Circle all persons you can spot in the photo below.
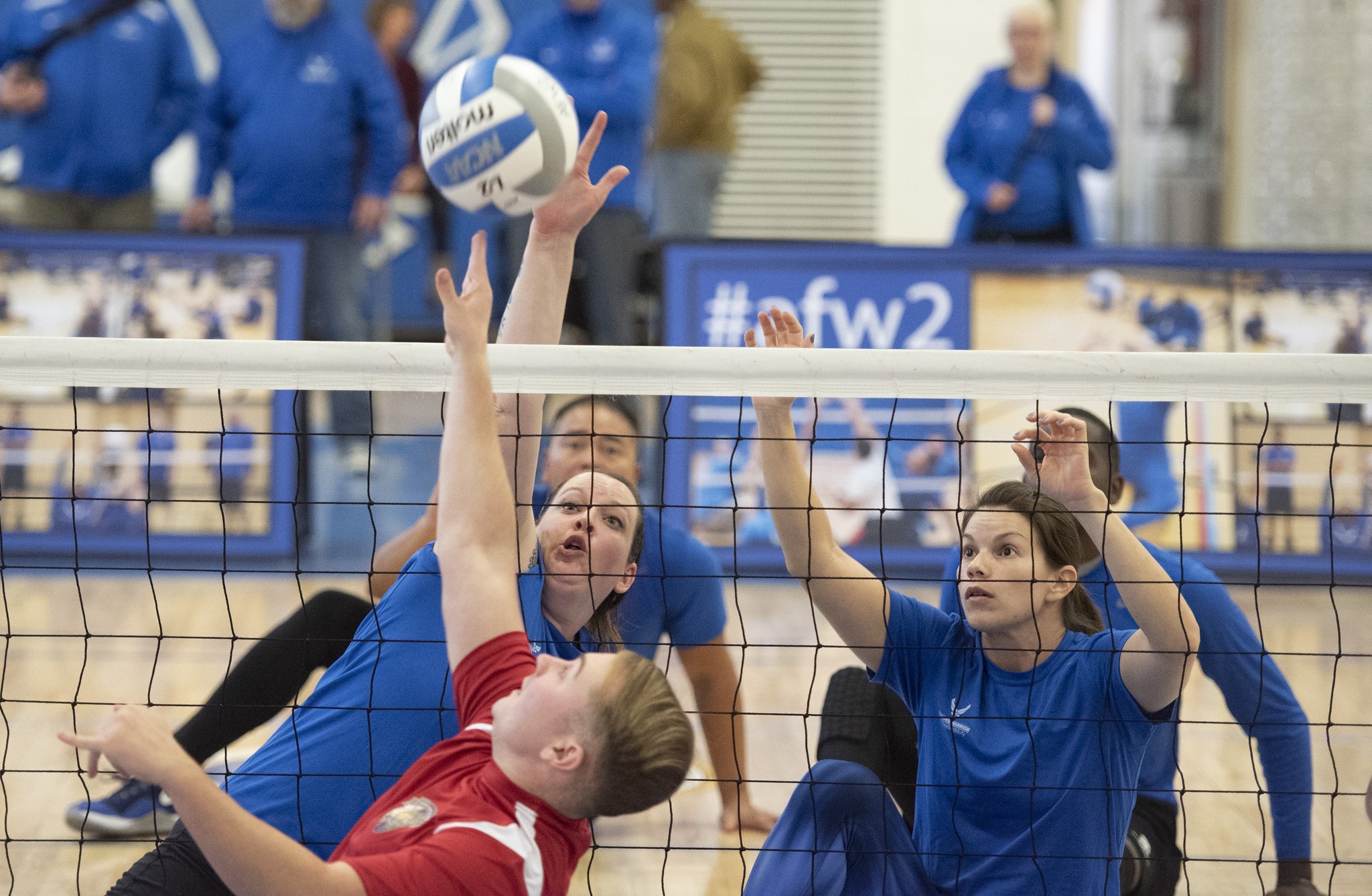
[0,256,262,538]
[945,0,1116,250]
[741,303,1321,896]
[56,109,779,896]
[0,0,769,474]
[681,259,1372,559]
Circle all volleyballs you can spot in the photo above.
[417,51,582,221]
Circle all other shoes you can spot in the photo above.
[339,440,378,475]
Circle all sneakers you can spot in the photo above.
[66,773,180,836]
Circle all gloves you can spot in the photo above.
[1266,859,1323,896]
